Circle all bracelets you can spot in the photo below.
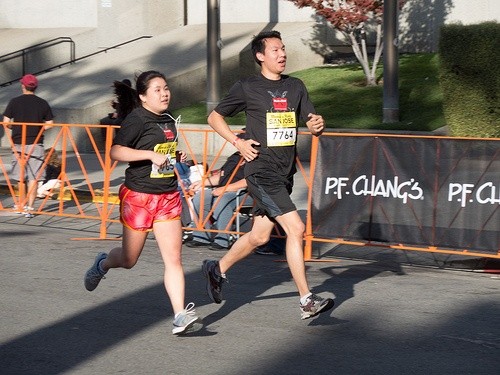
[232,137,239,146]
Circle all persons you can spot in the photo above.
[36,148,62,200]
[112,78,137,126]
[2,75,54,219]
[201,32,335,319]
[82,71,204,335]
[178,159,208,243]
[187,127,254,250]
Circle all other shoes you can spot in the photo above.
[185,240,229,251]
[51,194,58,200]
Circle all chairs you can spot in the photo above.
[196,168,281,240]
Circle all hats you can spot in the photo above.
[19,74,38,88]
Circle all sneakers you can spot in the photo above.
[84,252,109,291]
[11,205,35,218]
[202,259,226,304]
[171,302,204,335]
[256,246,278,254]
[299,294,335,320]
